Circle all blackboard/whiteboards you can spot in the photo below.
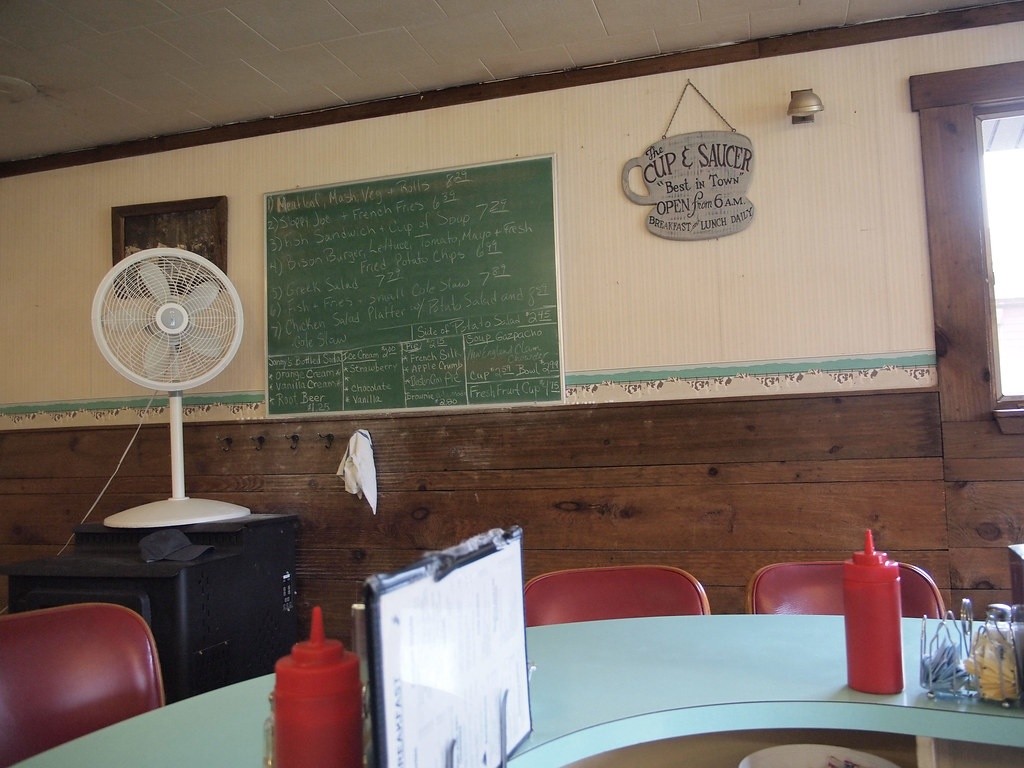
[264,153,567,415]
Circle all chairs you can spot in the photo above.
[746,561,946,620]
[522,565,711,627]
[0,604,165,768]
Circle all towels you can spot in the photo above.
[335,428,378,516]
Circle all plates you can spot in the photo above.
[738,745,902,768]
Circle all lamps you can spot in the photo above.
[786,88,824,125]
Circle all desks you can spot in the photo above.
[0,513,300,707]
[6,613,1024,768]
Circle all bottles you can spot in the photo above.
[975,606,1012,699]
[274,605,365,768]
[843,529,904,696]
[262,692,274,768]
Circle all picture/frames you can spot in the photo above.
[110,195,228,300]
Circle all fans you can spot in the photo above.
[90,247,252,529]
